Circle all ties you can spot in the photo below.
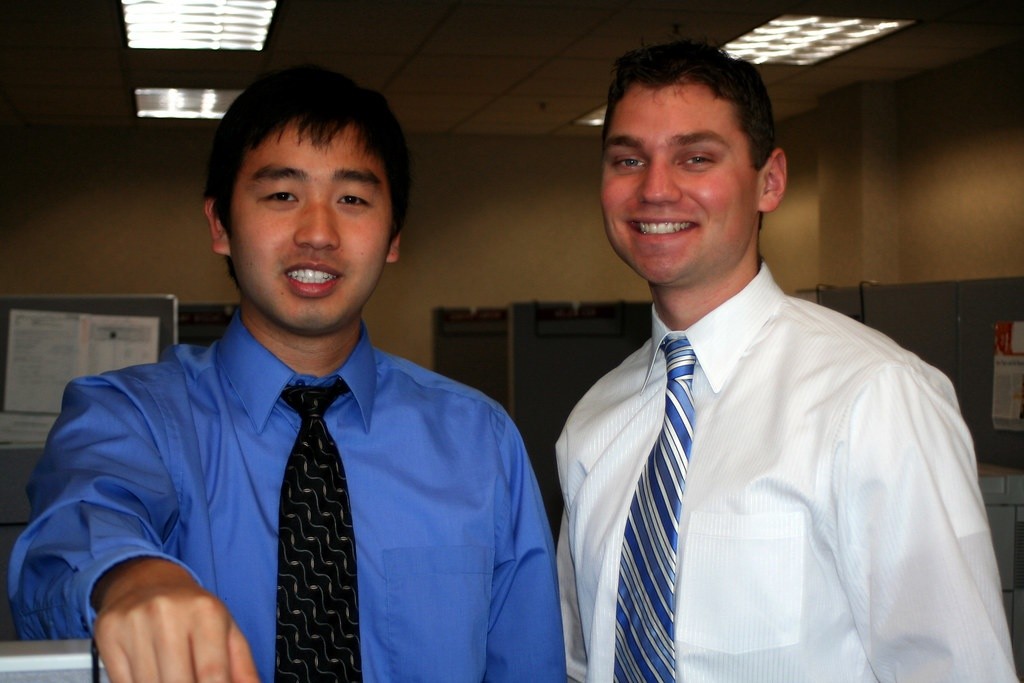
[274,379,364,683]
[613,336,697,683]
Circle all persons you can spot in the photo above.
[555,39,1021,683]
[3,64,567,683]
